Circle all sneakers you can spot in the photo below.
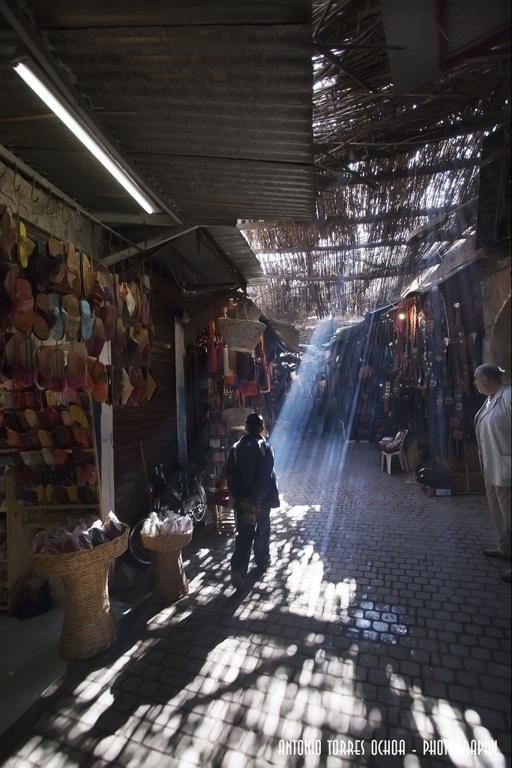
[230,568,241,585]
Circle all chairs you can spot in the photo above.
[380,428,410,475]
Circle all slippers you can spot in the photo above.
[0,203,170,505]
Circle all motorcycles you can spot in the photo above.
[126,463,211,567]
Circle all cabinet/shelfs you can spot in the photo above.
[1,341,101,619]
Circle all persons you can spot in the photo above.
[470,362,511,583]
[221,410,281,594]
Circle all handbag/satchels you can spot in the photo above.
[452,431,463,440]
[384,439,402,453]
[415,457,464,489]
[448,417,462,428]
[201,319,270,493]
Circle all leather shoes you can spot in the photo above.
[482,548,501,557]
[500,569,511,582]
